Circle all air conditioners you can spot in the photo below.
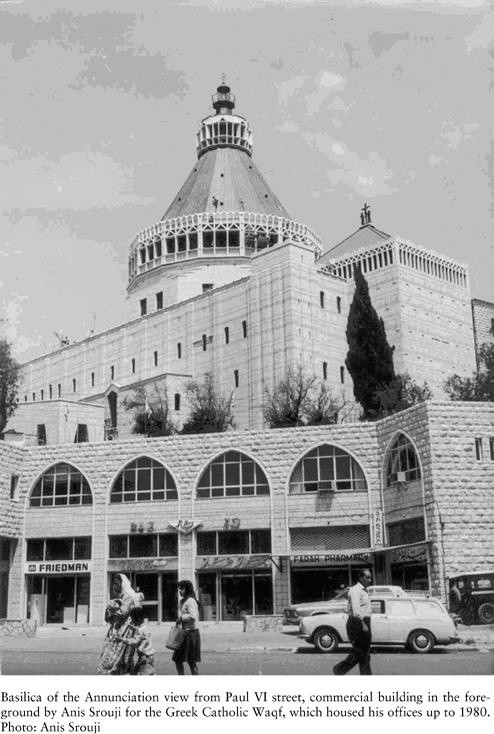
[389,472,405,484]
[318,481,334,492]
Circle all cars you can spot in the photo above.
[449,570,494,625]
[298,597,460,654]
[283,584,408,626]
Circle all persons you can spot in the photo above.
[171,579,199,676]
[112,607,157,675]
[332,567,372,674]
[96,599,134,674]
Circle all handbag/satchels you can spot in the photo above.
[166,623,186,651]
